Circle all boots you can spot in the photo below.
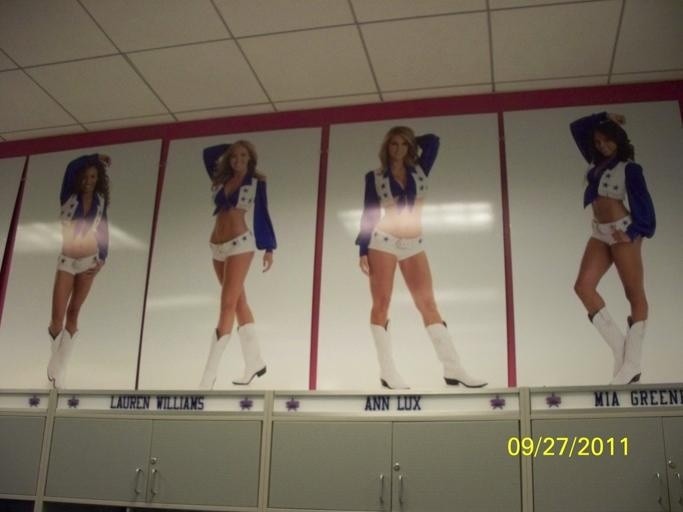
[233,323,267,385]
[370,320,411,390]
[45,328,80,387]
[198,327,231,389]
[589,306,646,385]
[427,322,488,388]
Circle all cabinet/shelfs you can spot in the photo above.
[45,417,264,510]
[268,419,524,512]
[531,416,680,510]
[3,414,48,498]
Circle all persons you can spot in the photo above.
[46,151,112,391]
[194,138,278,391]
[567,110,656,389]
[354,126,489,392]
